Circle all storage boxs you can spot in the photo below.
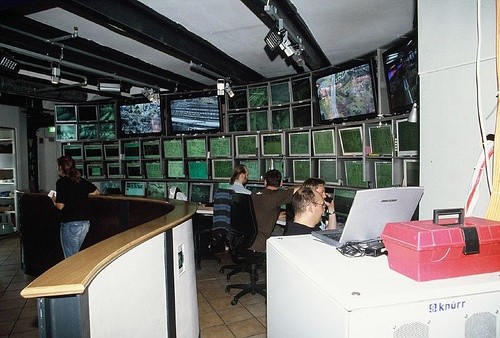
[381,208,500,281]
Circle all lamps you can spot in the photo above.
[0,55,20,77]
[97,78,122,94]
[50,62,60,84]
[217,78,234,97]
[264,19,305,67]
[408,102,420,123]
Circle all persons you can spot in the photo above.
[246,168,301,254]
[284,188,323,236]
[301,176,337,230]
[47,155,101,259]
[228,164,252,195]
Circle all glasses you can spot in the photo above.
[312,202,325,209]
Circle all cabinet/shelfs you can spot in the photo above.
[0,126,19,231]
[265,235,500,338]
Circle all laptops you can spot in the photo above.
[311,187,425,247]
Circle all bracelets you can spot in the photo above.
[327,211,336,215]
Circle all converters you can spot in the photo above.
[365,243,386,257]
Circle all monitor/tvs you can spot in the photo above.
[54,27,420,219]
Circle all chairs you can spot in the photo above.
[193,188,267,305]
